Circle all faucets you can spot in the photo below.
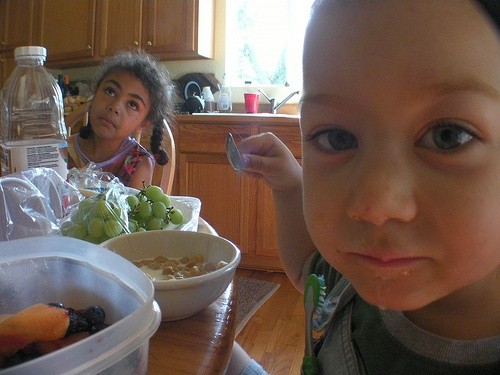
[271,90,300,115]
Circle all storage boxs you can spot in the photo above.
[0,232,163,374]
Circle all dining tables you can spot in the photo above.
[0,191,241,375]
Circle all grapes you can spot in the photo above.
[60,194,127,245]
[126,180,184,232]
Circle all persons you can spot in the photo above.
[225,0,500,375]
[67,54,169,190]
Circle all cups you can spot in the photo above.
[244,94,260,113]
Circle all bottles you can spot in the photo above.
[57,75,64,98]
[202,74,253,112]
[0,46,70,178]
[64,75,70,97]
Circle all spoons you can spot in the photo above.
[225,132,247,175]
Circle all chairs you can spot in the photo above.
[68,103,176,197]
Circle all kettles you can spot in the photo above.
[183,79,206,113]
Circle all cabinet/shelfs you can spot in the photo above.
[174,114,303,274]
[0,0,213,64]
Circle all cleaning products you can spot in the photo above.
[217,73,232,113]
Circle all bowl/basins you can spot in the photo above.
[99,230,242,319]
[1,237,162,375]
